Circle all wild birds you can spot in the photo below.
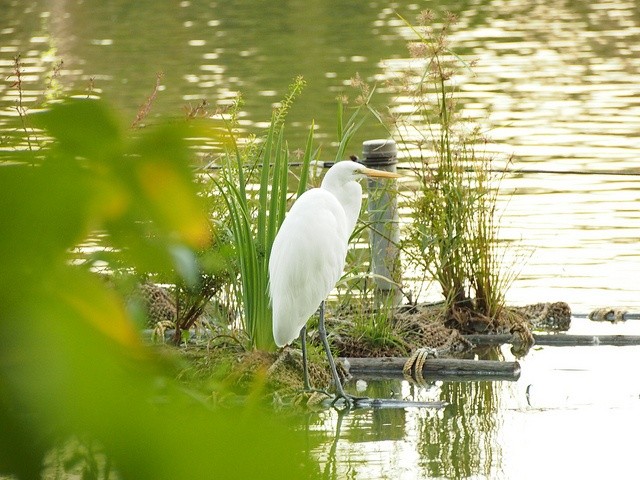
[260,157,402,410]
